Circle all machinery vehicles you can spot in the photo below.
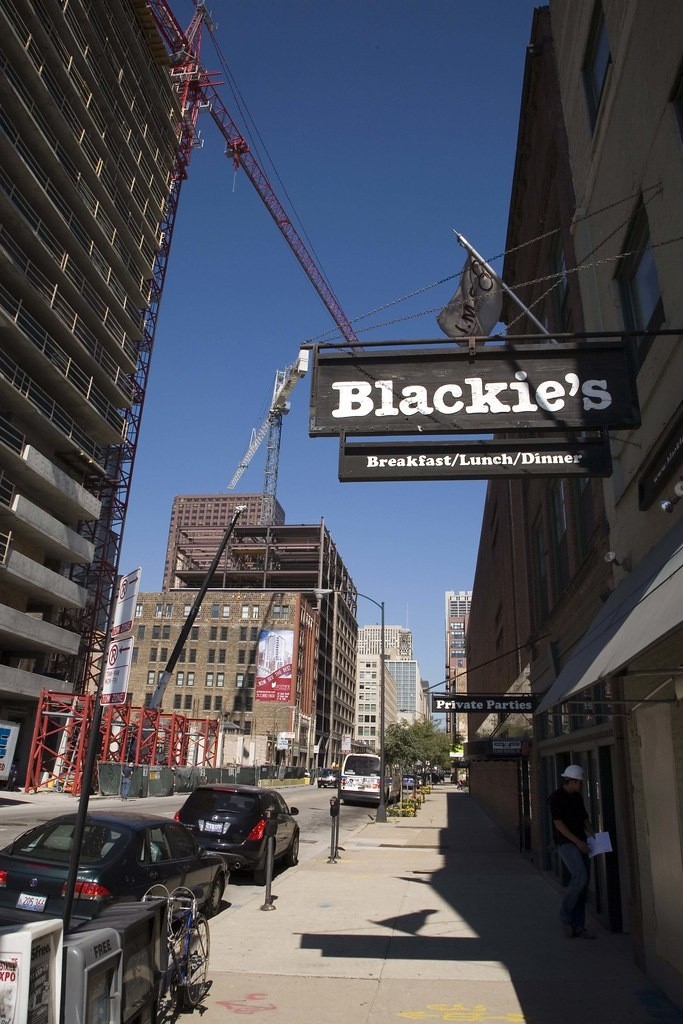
[121,504,247,764]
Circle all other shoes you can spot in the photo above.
[573,928,597,939]
[559,909,571,928]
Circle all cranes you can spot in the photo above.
[65,1,368,771]
[226,342,317,571]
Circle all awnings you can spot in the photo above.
[533,724,616,756]
[535,532,682,714]
[452,761,470,768]
[464,740,529,763]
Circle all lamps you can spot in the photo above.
[659,494,681,513]
[602,550,629,571]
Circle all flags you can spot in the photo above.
[438,257,506,348]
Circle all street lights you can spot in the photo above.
[313,589,389,823]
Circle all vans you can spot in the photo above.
[339,752,400,807]
[317,768,339,788]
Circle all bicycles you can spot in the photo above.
[141,882,211,1024]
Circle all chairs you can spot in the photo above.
[47,835,74,853]
[141,838,162,861]
[100,829,122,858]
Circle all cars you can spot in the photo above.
[418,773,440,786]
[401,773,420,790]
[0,810,231,931]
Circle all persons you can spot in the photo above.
[548,765,596,939]
[121,762,134,802]
[342,777,380,789]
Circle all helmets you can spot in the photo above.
[561,765,586,781]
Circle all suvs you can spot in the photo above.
[173,783,301,883]
